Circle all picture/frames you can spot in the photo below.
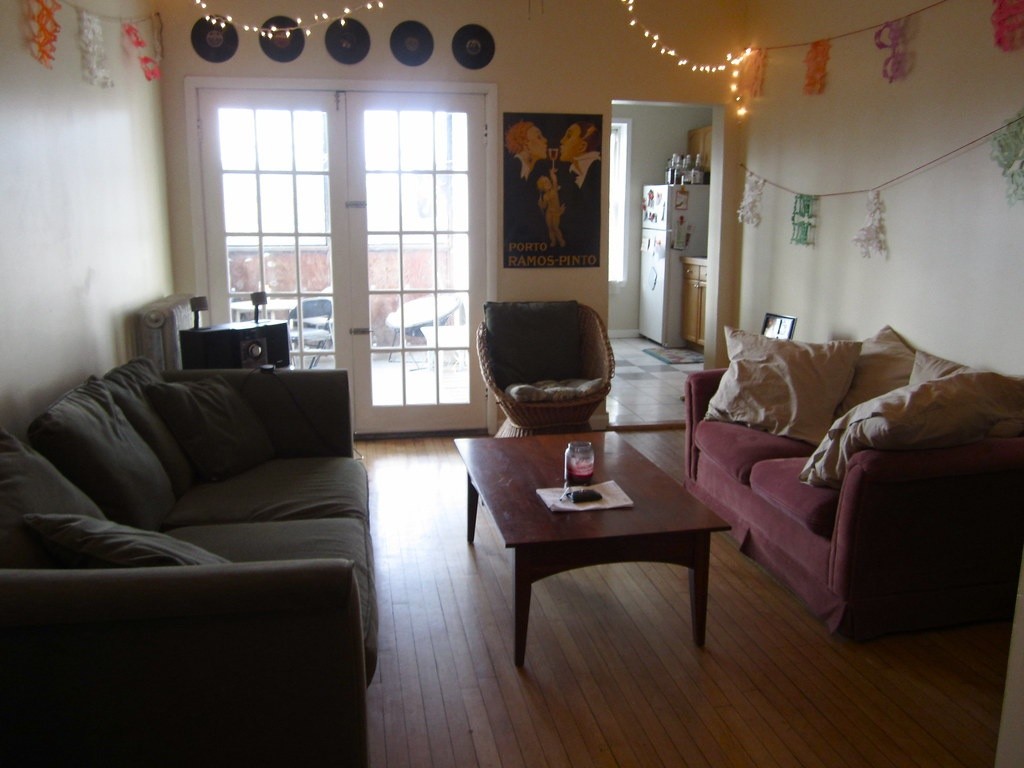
[761,312,797,341]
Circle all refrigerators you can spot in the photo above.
[639,184,710,348]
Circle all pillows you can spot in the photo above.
[0,354,277,569]
[484,300,587,391]
[700,324,1024,488]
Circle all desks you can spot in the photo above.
[454,430,732,668]
[231,299,305,323]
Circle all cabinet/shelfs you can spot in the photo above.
[687,125,712,172]
[681,262,707,354]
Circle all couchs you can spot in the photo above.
[1,368,379,767]
[683,367,1024,641]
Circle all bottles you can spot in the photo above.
[665,152,704,184]
[563,440,595,483]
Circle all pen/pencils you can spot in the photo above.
[559,487,570,501]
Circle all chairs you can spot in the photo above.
[385,292,460,367]
[288,299,332,369]
[475,303,615,438]
